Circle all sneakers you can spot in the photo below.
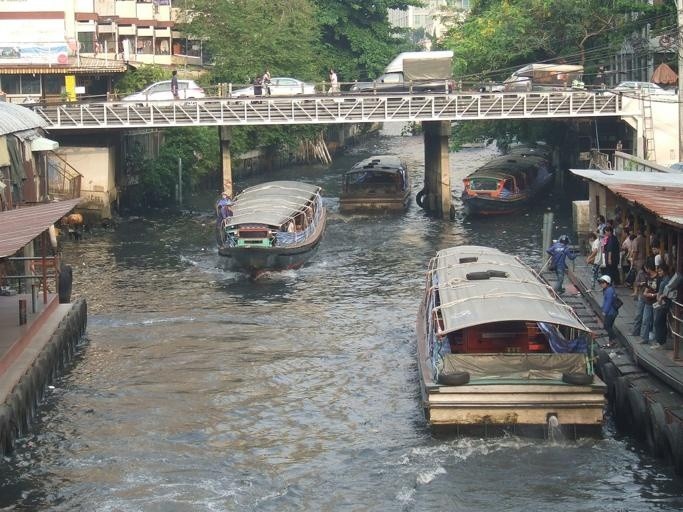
[649,343,665,350]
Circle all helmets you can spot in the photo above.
[596,275,610,284]
[559,235,569,244]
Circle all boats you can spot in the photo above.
[340,154,410,210]
[217,181,327,258]
[462,143,552,214]
[416,246,609,432]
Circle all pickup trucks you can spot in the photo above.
[480,76,588,98]
[348,71,455,94]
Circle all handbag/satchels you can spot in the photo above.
[611,294,622,310]
[547,262,557,271]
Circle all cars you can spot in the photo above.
[121,79,206,107]
[229,78,316,99]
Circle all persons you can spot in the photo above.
[325,69,337,96]
[170,69,180,100]
[213,192,232,221]
[544,233,583,293]
[584,205,682,350]
[252,74,262,104]
[262,68,272,97]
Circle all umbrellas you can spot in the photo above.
[649,62,677,85]
